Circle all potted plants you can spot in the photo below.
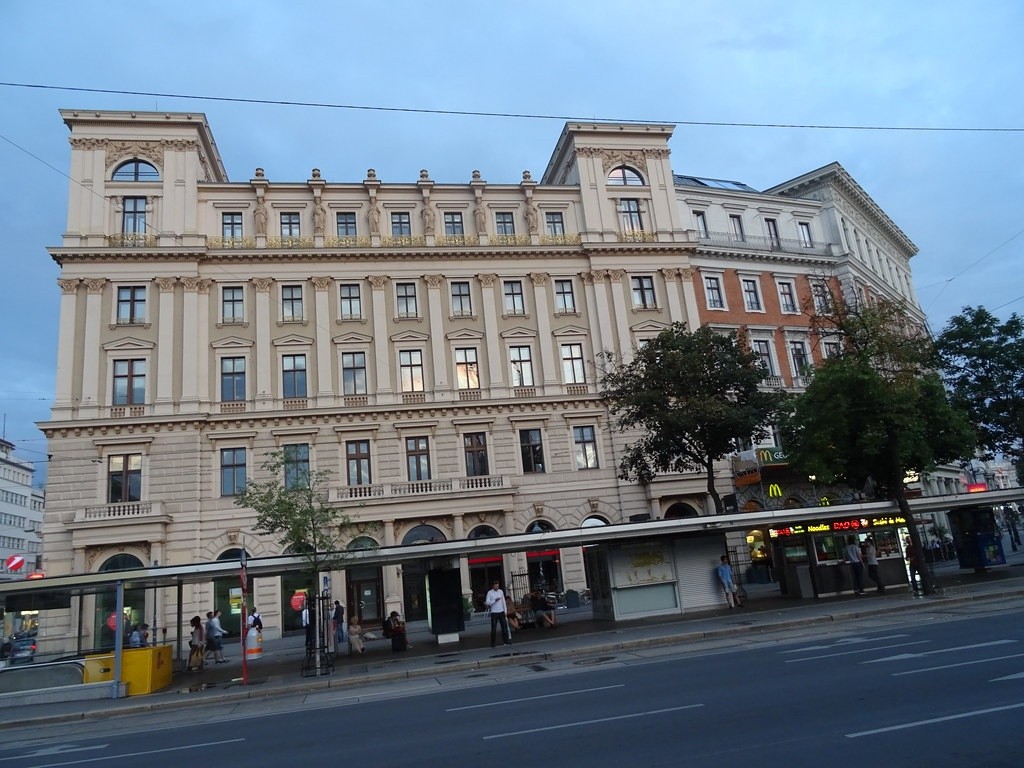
[462,595,476,622]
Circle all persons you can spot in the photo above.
[349,617,367,656]
[330,600,344,642]
[864,538,885,592]
[505,596,523,632]
[927,529,957,562]
[486,581,512,648]
[129,624,150,647]
[846,535,866,595]
[302,608,309,628]
[246,607,263,631]
[749,545,766,560]
[183,609,228,674]
[527,591,560,629]
[717,555,744,609]
[904,536,922,592]
[382,611,414,650]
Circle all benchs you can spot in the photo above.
[345,626,407,657]
[508,605,559,632]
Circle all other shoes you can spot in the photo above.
[860,592,868,595]
[406,644,413,649]
[215,660,224,664]
[223,657,230,662]
[503,641,511,645]
[203,660,208,665]
[855,590,859,595]
[361,646,364,652]
[910,591,918,596]
[183,668,191,672]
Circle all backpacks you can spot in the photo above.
[251,615,263,629]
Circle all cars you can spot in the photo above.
[10,638,36,664]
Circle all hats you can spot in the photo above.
[391,611,399,617]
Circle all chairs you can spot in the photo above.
[483,602,491,618]
